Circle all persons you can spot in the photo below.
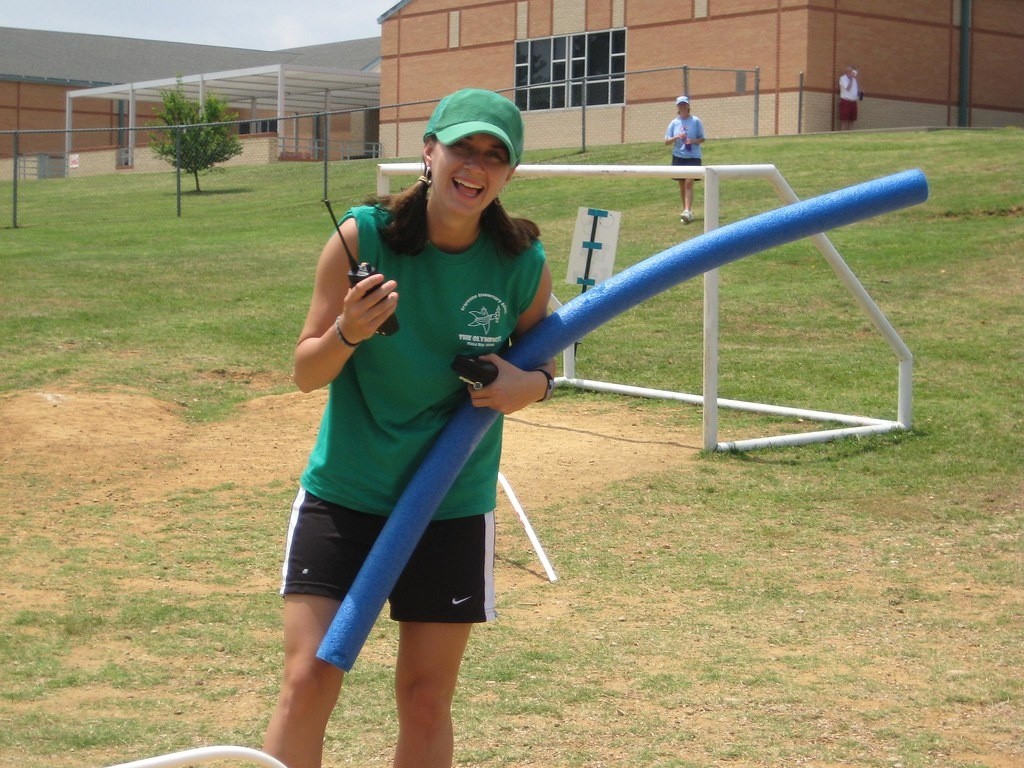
[263,89,556,767]
[839,65,864,131]
[665,96,705,223]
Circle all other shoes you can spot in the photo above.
[680,211,691,224]
[681,215,693,223]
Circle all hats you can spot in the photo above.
[676,96,690,105]
[422,87,524,167]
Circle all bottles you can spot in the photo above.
[860,91,863,101]
[683,137,691,151]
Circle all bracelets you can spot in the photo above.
[335,316,364,347]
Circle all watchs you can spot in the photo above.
[536,369,554,402]
